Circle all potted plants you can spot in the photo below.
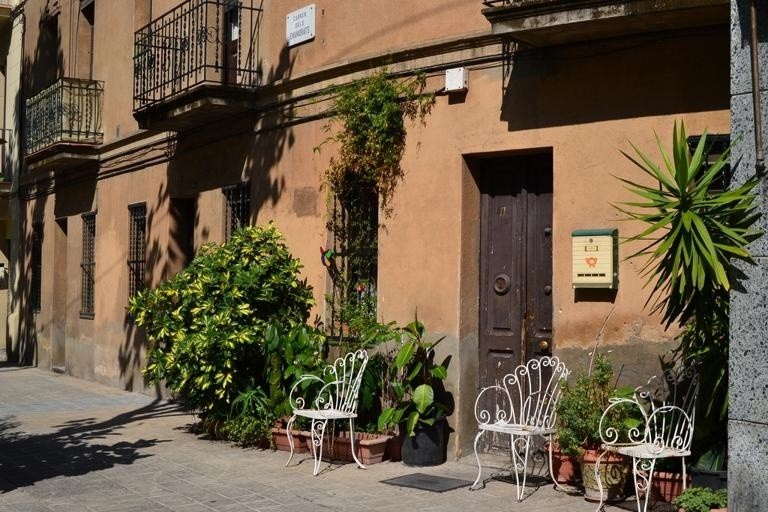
[302,353,390,464]
[124,221,318,440]
[546,378,589,488]
[610,119,764,504]
[578,352,640,502]
[376,304,447,466]
[271,322,332,452]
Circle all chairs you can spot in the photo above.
[283,348,369,476]
[469,355,572,502]
